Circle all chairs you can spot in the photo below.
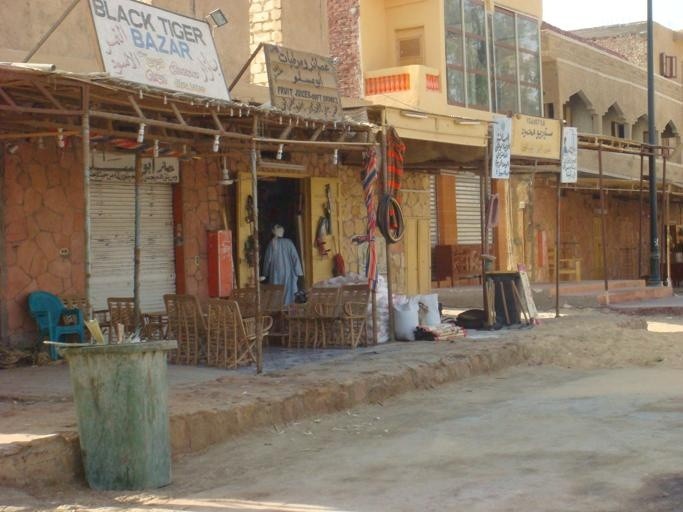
[23,289,113,362]
[556,241,584,282]
[106,282,373,367]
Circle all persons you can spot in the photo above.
[258,225,305,306]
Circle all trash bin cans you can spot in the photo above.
[67,341,179,491]
[489,273,521,323]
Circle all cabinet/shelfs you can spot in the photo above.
[433,244,488,288]
